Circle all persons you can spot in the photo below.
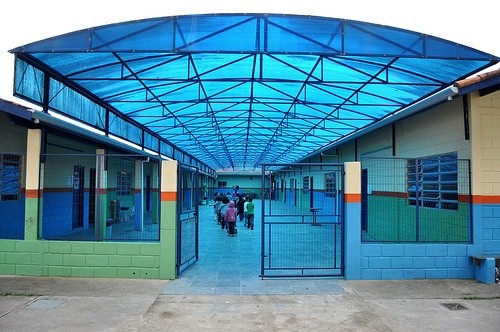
[213,192,254,237]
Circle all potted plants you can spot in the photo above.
[199,184,208,205]
[107,218,112,239]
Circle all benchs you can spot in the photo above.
[469,255,500,285]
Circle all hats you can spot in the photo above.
[228,201,235,206]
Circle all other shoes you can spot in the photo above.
[251,227,253,230]
[227,233,230,236]
[244,224,246,227]
[247,226,250,228]
[232,234,235,237]
[222,227,224,229]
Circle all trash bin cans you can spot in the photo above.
[206,198,209,205]
[106,218,113,240]
[120,207,129,222]
